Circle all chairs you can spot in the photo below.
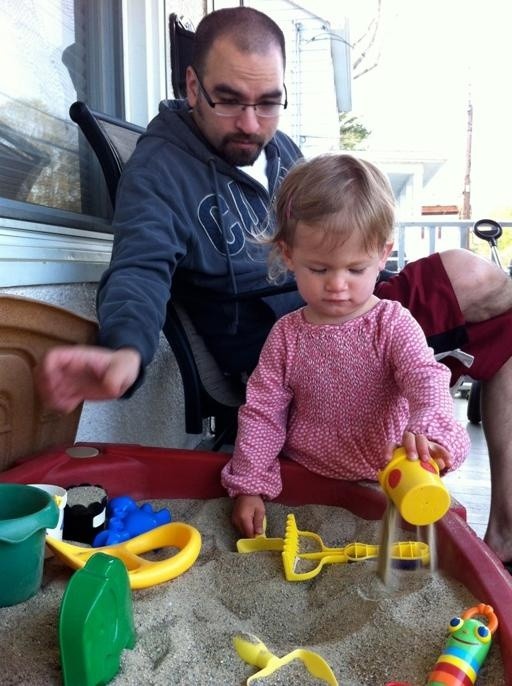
[69,102,278,432]
[2,119,48,201]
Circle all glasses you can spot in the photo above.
[190,67,288,119]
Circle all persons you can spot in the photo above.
[38,5,510,571]
[223,144,471,542]
